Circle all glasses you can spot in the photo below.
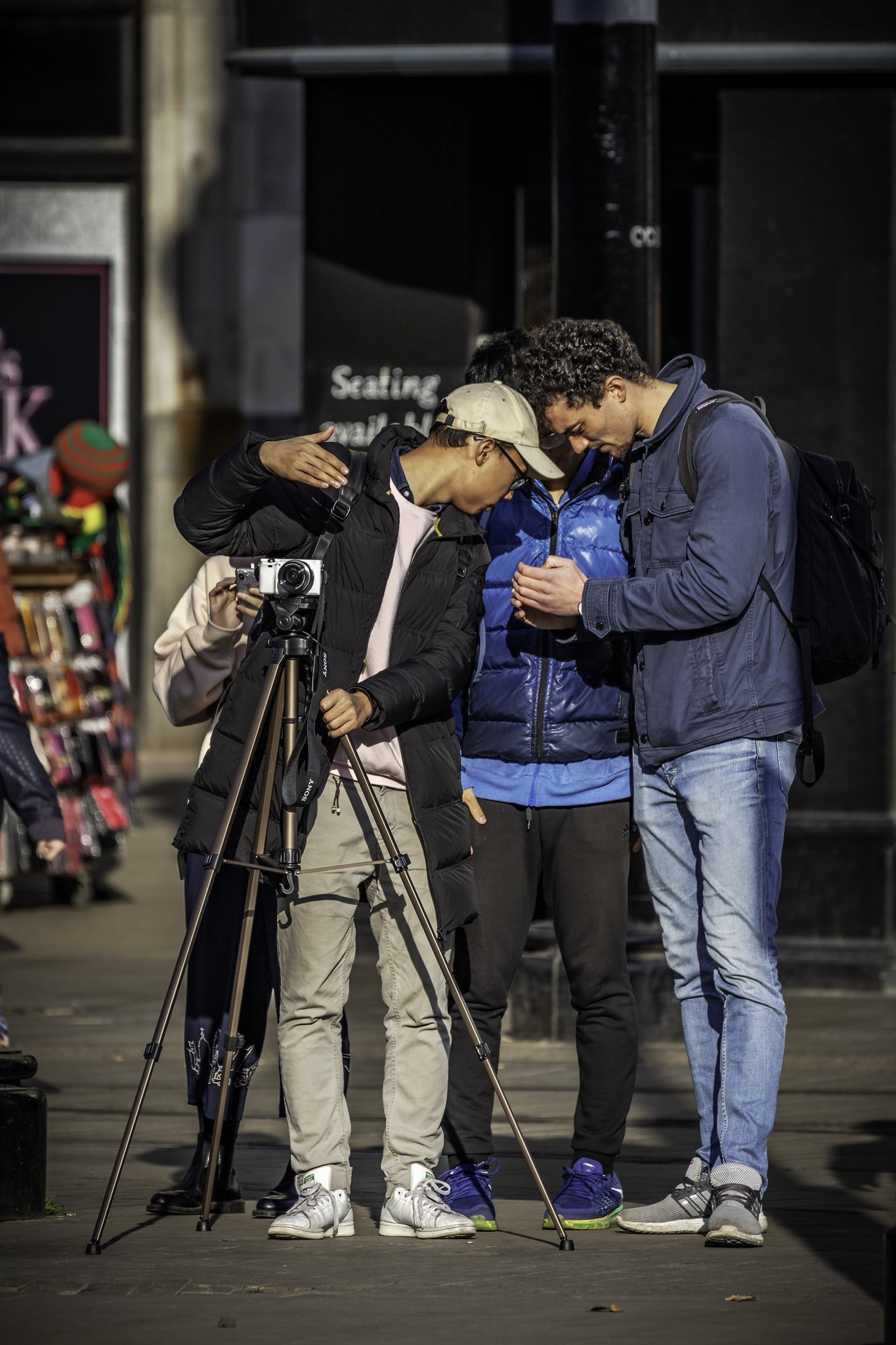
[536,432,567,449]
[472,435,531,490]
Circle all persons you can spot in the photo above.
[7,419,139,645]
[505,314,828,1249]
[144,555,351,1218]
[172,378,565,1239]
[0,631,69,1054]
[431,329,636,1234]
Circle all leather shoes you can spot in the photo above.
[252,1154,303,1220]
[146,1133,244,1216]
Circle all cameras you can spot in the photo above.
[255,559,325,597]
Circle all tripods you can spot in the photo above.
[80,600,574,1253]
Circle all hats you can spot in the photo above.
[434,380,567,479]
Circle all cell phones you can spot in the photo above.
[234,567,259,607]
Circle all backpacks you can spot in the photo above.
[677,385,892,787]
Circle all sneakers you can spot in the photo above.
[542,1158,624,1230]
[704,1163,765,1248]
[378,1164,475,1240]
[616,1155,768,1235]
[267,1165,355,1239]
[438,1164,498,1231]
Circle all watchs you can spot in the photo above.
[577,601,582,615]
